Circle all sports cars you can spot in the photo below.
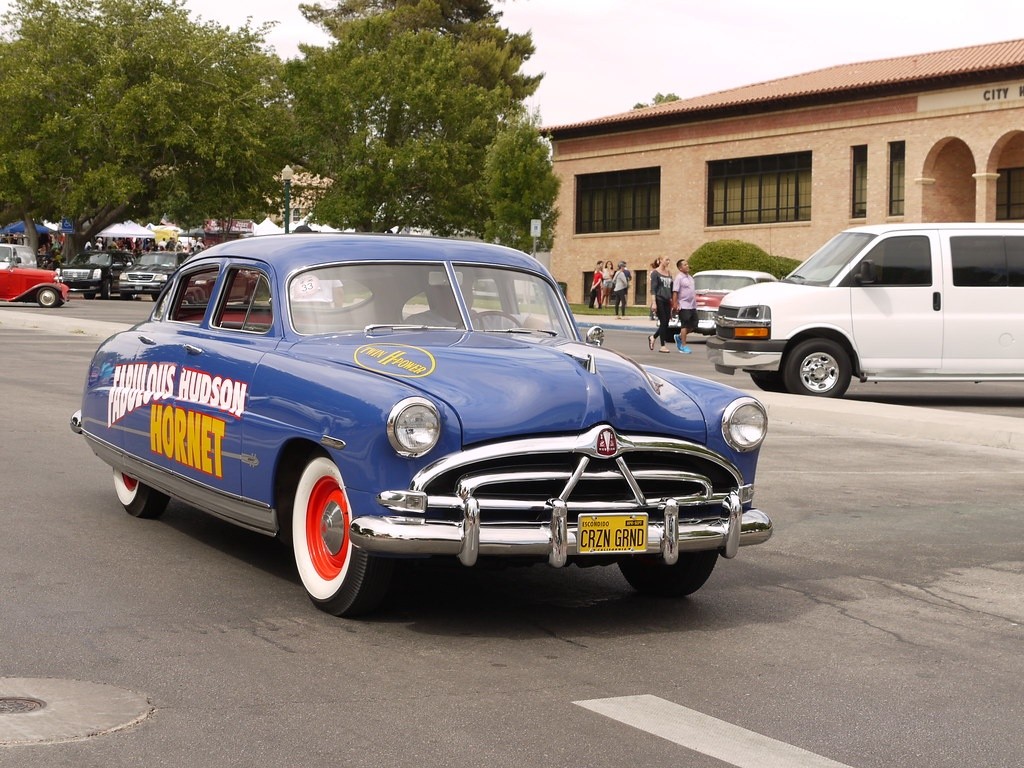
[0,261,72,309]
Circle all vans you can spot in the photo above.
[706,222,1024,399]
[0,243,37,272]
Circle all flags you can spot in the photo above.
[161,214,177,226]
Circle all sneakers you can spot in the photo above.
[679,346,691,353]
[674,334,681,350]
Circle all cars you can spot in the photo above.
[179,266,270,307]
[656,269,779,343]
[70,232,774,616]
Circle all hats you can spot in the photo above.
[96,238,102,241]
[197,237,202,240]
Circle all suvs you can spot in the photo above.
[54,246,138,300]
[118,249,193,302]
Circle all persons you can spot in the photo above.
[0,235,212,257]
[402,271,549,330]
[649,255,680,355]
[589,260,632,321]
[672,259,699,353]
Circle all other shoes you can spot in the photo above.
[658,346,670,353]
[614,316,621,319]
[648,335,655,350]
[621,316,630,320]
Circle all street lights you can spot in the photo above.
[282,165,293,233]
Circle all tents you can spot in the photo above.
[252,217,359,234]
[1,221,52,233]
[95,219,157,238]
